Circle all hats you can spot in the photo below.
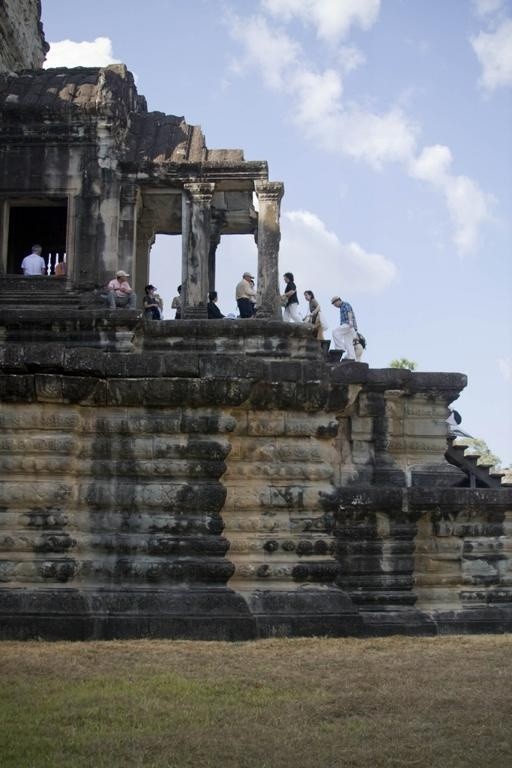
[242,271,255,279]
[331,296,340,304]
[117,269,130,278]
[224,313,236,319]
[145,284,157,293]
[209,291,217,300]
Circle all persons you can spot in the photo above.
[235,272,257,317]
[143,284,164,321]
[303,291,326,340]
[106,269,136,311]
[280,273,303,322]
[208,291,225,319]
[170,285,183,319]
[20,243,47,277]
[54,261,67,275]
[331,295,358,362]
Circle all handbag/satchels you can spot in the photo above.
[303,315,312,324]
[353,340,364,361]
[152,309,160,320]
[357,331,366,349]
[280,295,289,307]
[315,311,328,331]
